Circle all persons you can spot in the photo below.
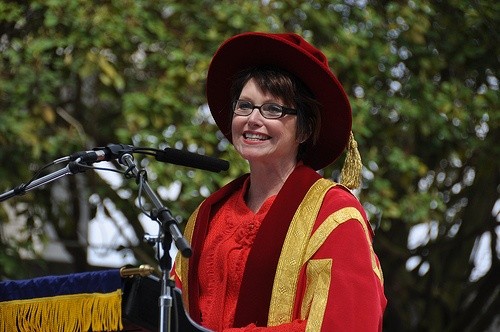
[168,32,388,332]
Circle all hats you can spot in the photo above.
[205,32,364,190]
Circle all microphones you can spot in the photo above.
[35,145,230,174]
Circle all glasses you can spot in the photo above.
[232,98,297,121]
